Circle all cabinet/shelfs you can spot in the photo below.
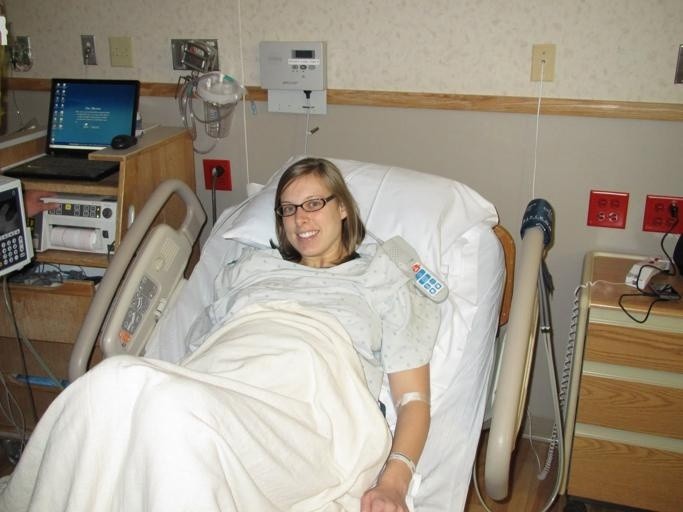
[0,123,199,442]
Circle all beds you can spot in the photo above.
[0,155,555,512]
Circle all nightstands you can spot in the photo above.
[555,251,683,511]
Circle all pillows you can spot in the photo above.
[223,155,499,284]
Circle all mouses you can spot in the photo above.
[111,134,138,150]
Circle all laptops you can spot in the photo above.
[3,76,141,182]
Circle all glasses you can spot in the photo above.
[274,195,336,217]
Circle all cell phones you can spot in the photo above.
[649,280,681,301]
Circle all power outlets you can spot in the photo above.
[202,158,232,192]
[588,191,629,229]
[643,195,683,235]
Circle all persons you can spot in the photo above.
[1,157,431,512]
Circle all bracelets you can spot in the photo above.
[387,452,415,478]
